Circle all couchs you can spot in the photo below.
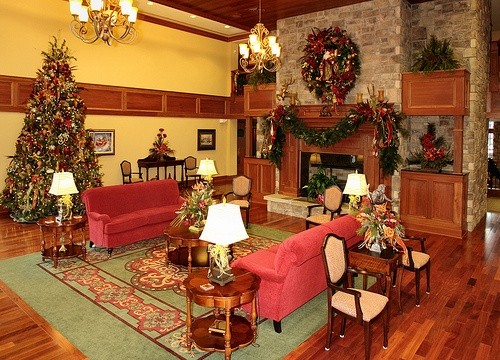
[82,179,189,257]
[231,216,365,333]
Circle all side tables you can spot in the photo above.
[182,269,261,360]
[38,215,88,269]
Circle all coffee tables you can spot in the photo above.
[163,224,236,273]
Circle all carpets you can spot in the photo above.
[0,224,380,360]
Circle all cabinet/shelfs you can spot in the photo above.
[400,69,470,239]
[243,83,276,204]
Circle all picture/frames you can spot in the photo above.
[197,129,216,151]
[92,130,115,155]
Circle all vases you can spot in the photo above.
[188,224,205,234]
[157,153,164,161]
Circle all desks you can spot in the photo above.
[135,160,185,187]
[346,241,406,332]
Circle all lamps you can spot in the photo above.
[201,203,250,284]
[67,0,139,45]
[239,0,282,73]
[195,157,219,184]
[344,169,369,196]
[50,169,80,221]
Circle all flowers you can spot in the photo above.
[296,25,359,106]
[149,127,177,156]
[351,200,405,252]
[416,124,453,168]
[171,180,217,225]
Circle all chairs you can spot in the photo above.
[185,156,201,187]
[304,184,344,229]
[223,176,252,229]
[120,160,143,184]
[393,234,434,309]
[321,233,391,360]
